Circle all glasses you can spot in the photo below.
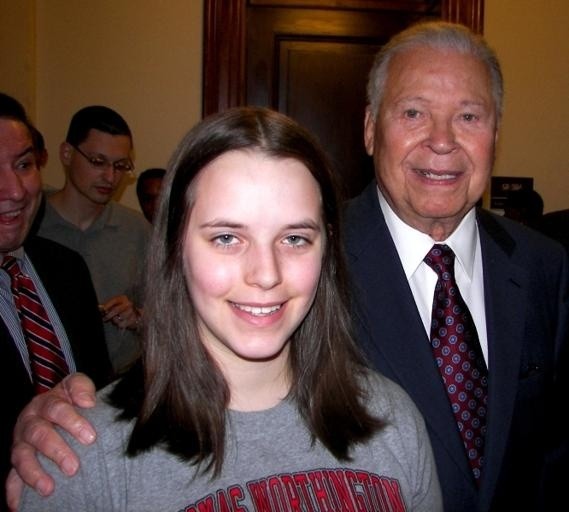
[71,143,134,173]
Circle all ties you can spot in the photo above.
[423,246,489,490]
[0,256,70,400]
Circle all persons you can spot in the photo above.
[2,18,569,511]
[0,91,116,512]
[135,168,167,226]
[37,102,154,376]
[8,105,446,511]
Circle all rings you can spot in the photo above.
[116,314,124,322]
